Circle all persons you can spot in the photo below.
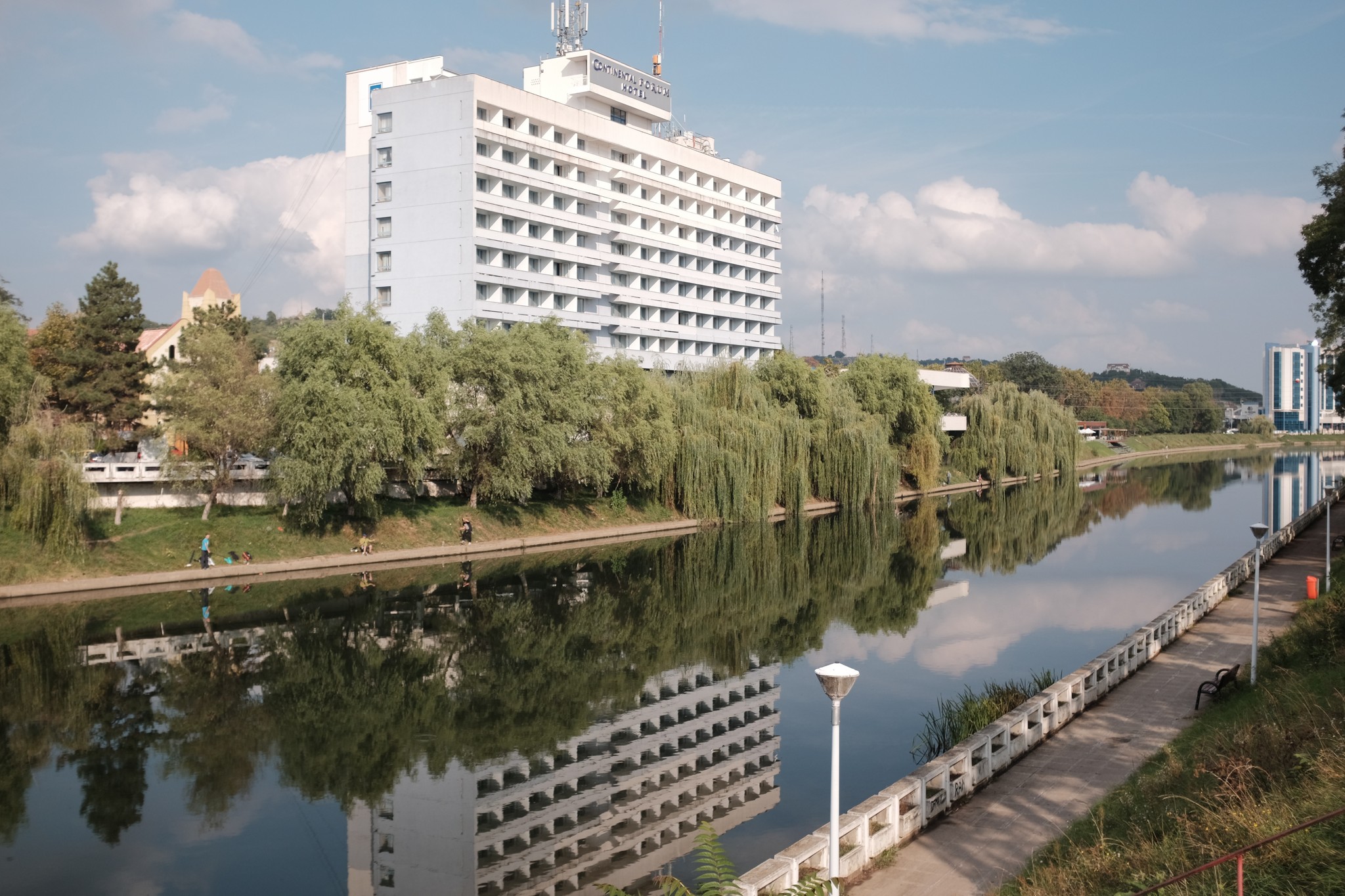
[947,471,952,485]
[977,490,982,498]
[201,588,211,622]
[360,534,375,556]
[360,572,375,591]
[460,561,472,586]
[242,584,251,593]
[242,552,252,565]
[946,494,951,508]
[201,534,211,570]
[459,518,473,545]
[978,474,982,482]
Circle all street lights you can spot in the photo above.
[1322,484,1336,595]
[814,661,859,896]
[1226,408,1233,433]
[1249,522,1270,688]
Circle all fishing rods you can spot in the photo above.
[210,551,265,575]
[456,499,463,538]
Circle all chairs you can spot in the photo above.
[1332,535,1345,552]
[1194,663,1240,709]
